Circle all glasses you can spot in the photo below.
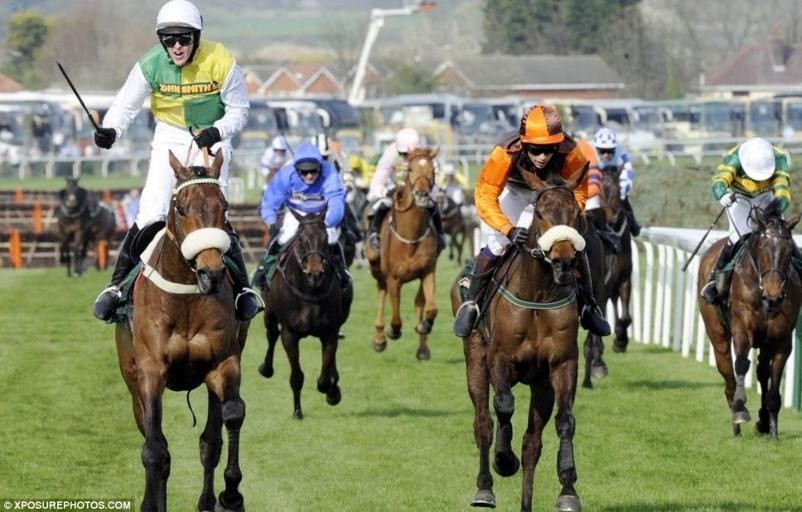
[599,150,613,154]
[525,146,561,154]
[161,35,192,46]
[297,169,318,175]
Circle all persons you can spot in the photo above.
[92,0,259,319]
[259,103,633,337]
[701,138,791,304]
[31,103,99,155]
[122,188,141,229]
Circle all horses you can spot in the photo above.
[581,159,634,392]
[364,143,441,363]
[81,199,118,258]
[112,145,253,512]
[696,197,802,445]
[447,159,594,512]
[257,198,355,420]
[56,175,93,279]
[439,193,467,267]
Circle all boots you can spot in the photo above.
[251,235,281,309]
[369,202,391,251]
[453,248,497,338]
[328,241,352,293]
[701,241,737,304]
[94,222,141,320]
[426,202,445,248]
[572,250,611,336]
[218,220,258,321]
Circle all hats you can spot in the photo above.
[295,161,320,170]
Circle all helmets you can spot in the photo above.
[594,128,617,149]
[156,0,203,34]
[738,138,776,182]
[397,127,419,154]
[519,105,566,146]
[272,135,287,151]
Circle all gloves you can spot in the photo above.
[268,223,280,236]
[94,127,117,150]
[720,193,732,207]
[588,208,607,227]
[508,226,530,253]
[193,126,222,150]
